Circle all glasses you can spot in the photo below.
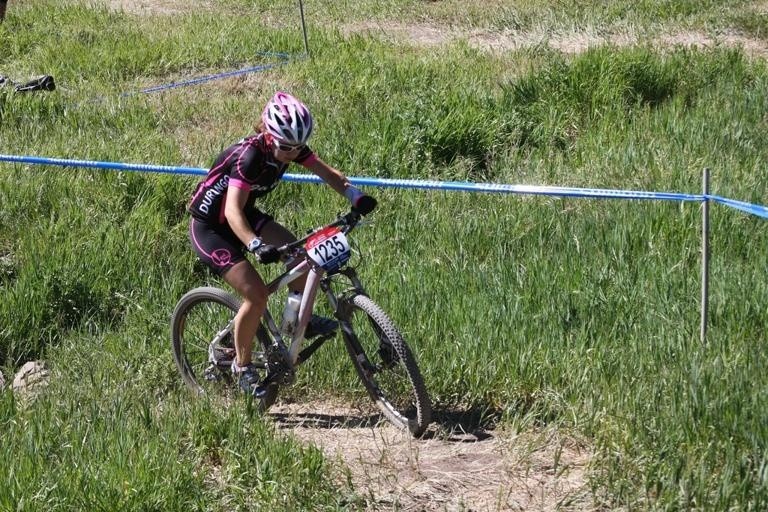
[272,137,305,153]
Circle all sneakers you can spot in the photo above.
[230,356,267,397]
[284,315,339,339]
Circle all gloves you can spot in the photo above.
[356,195,378,216]
[255,244,279,264]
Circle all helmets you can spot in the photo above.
[261,92,313,144]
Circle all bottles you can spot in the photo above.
[280,291,303,338]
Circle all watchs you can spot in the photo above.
[248,236,263,253]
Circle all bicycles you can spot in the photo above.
[170,206,431,437]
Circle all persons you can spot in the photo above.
[186,91,378,397]
